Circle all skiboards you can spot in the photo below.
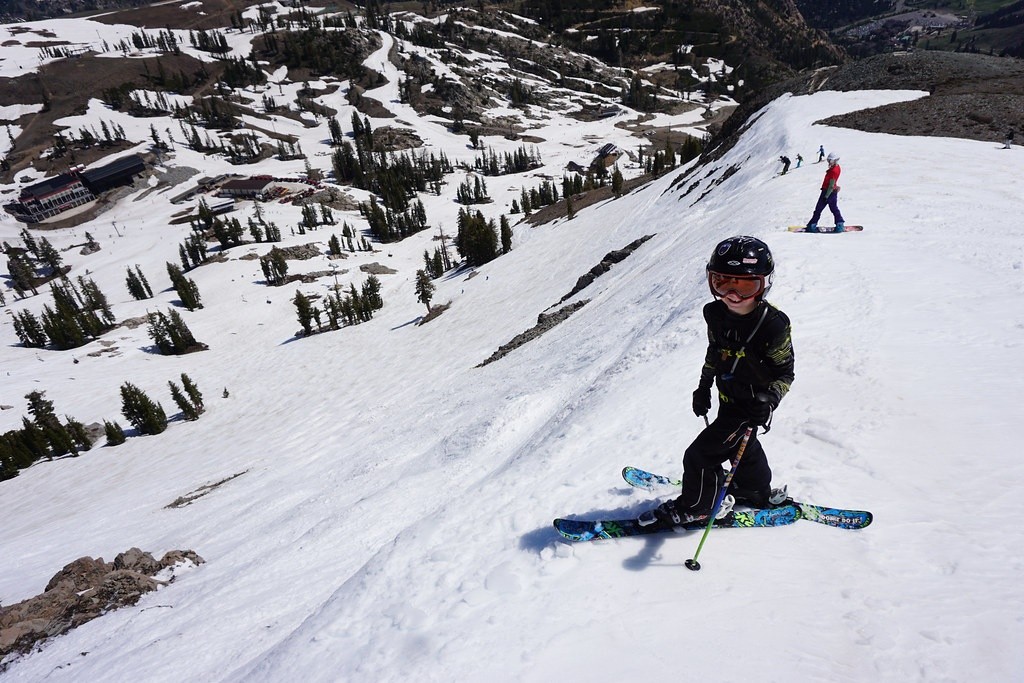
[771,172,791,178]
[552,466,873,542]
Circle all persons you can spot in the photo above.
[796,154,802,168]
[817,145,824,162]
[637,235,795,528]
[806,152,845,232]
[779,156,791,175]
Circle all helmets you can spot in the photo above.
[705,235,776,307]
[826,151,840,167]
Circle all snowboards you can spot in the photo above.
[787,225,863,234]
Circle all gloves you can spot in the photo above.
[749,386,781,426]
[692,386,713,417]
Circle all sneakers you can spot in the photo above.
[723,481,772,506]
[656,495,713,520]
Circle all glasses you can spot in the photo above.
[707,272,765,301]
